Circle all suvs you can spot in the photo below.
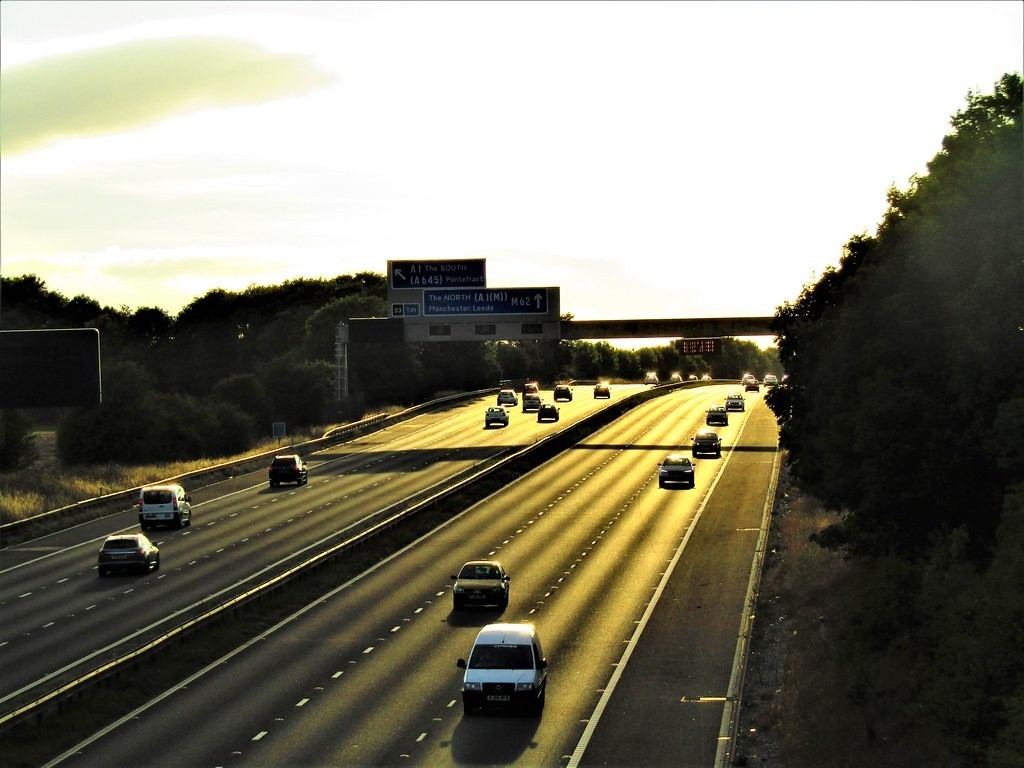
[97,533,160,579]
[763,375,778,387]
[725,393,744,411]
[268,453,308,487]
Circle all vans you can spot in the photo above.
[458,623,549,719]
[138,485,192,529]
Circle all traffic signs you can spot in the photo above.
[423,289,548,315]
[390,260,486,289]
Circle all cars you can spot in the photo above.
[658,454,695,488]
[668,371,713,384]
[538,403,560,422]
[745,379,760,392]
[690,431,722,458]
[643,372,659,384]
[485,406,510,427]
[705,405,729,426]
[497,389,519,406]
[522,392,544,412]
[553,385,574,401]
[451,558,510,613]
[522,383,540,398]
[594,384,612,398]
[742,375,755,386]
